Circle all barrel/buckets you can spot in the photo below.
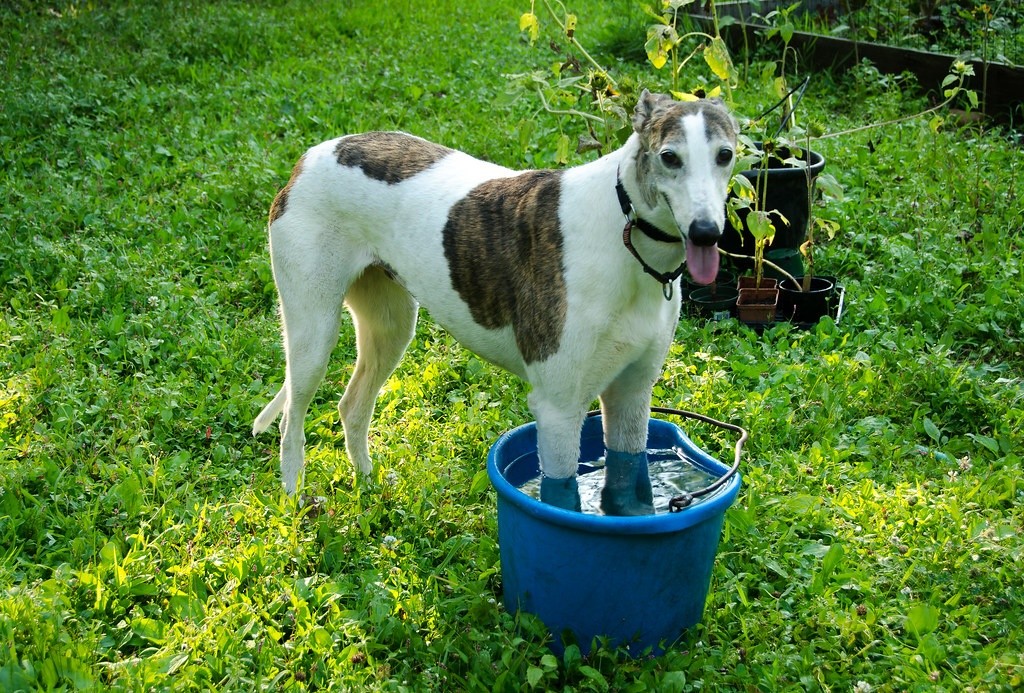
[487,407,748,665]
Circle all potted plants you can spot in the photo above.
[520,0,982,328]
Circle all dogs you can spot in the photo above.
[251,89,741,503]
